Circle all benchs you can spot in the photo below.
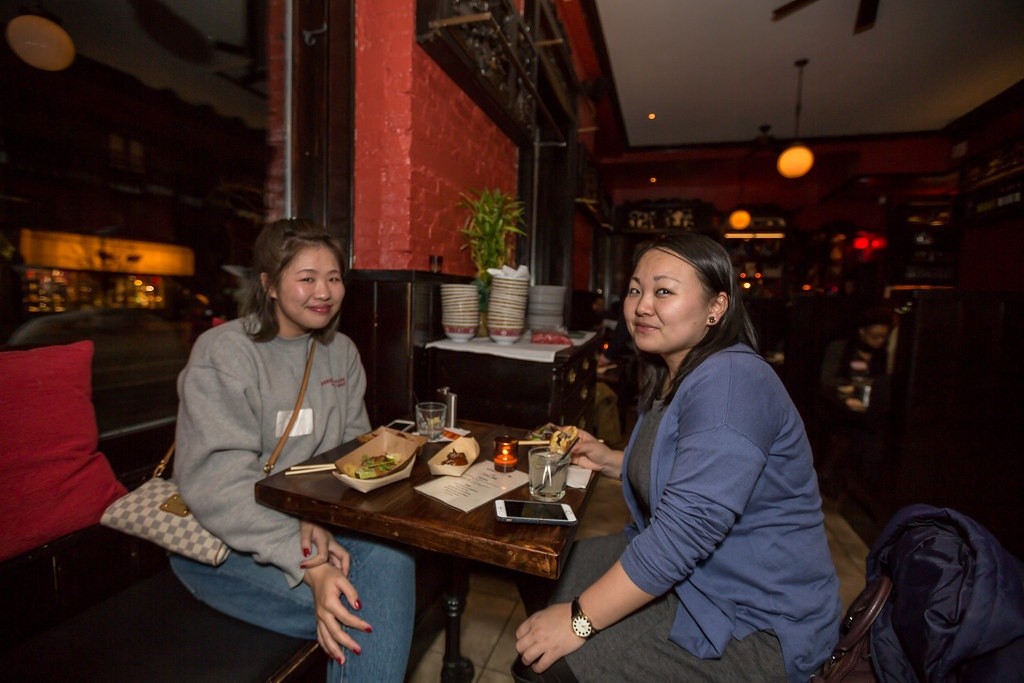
[833,294,1024,560]
[0,418,446,683]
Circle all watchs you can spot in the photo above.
[570,595,601,641]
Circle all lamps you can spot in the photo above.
[728,171,751,231]
[777,58,815,178]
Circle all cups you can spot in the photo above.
[493,436,519,472]
[430,255,442,273]
[415,402,447,440]
[528,447,571,503]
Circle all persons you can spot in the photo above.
[165,217,418,683]
[813,302,892,497]
[512,231,841,683]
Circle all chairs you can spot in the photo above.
[819,339,848,384]
[864,502,1024,683]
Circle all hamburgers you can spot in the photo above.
[549,426,578,454]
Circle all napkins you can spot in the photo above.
[537,452,592,489]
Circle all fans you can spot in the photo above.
[769,0,880,35]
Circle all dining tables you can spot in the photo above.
[815,372,890,427]
[254,416,599,683]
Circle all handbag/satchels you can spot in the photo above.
[100,476,231,567]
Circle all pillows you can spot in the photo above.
[0,340,131,557]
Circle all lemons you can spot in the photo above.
[386,454,401,465]
[354,470,377,480]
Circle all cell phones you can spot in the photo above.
[494,499,578,526]
[386,419,416,432]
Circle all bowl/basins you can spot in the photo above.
[441,283,479,342]
[486,277,530,344]
[527,284,566,330]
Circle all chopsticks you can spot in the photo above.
[517,439,604,445]
[285,463,336,476]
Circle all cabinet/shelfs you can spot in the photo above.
[427,332,601,433]
[895,203,960,286]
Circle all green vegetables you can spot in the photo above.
[361,454,393,469]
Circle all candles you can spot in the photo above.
[492,435,518,473]
